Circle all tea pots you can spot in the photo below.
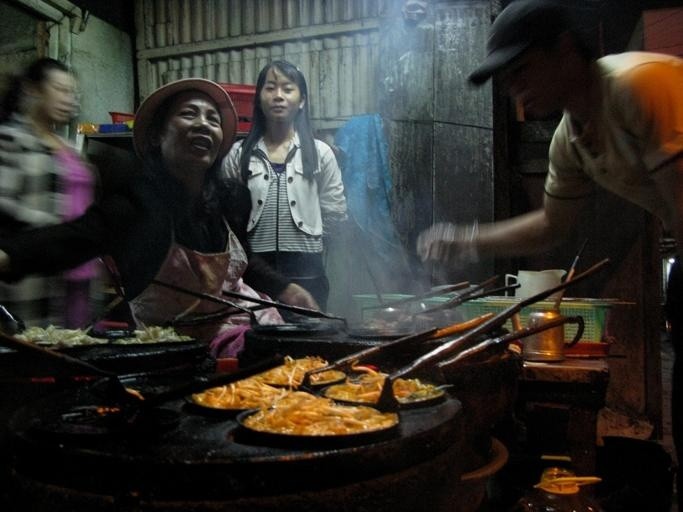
[505,270,584,362]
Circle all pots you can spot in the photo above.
[145,274,522,338]
[30,338,506,435]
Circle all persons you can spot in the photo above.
[0,57,104,331]
[0,77,321,332]
[416,0,683,512]
[221,59,347,315]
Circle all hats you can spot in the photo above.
[467,0,588,89]
[133,78,238,164]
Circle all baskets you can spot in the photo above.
[352,293,615,342]
